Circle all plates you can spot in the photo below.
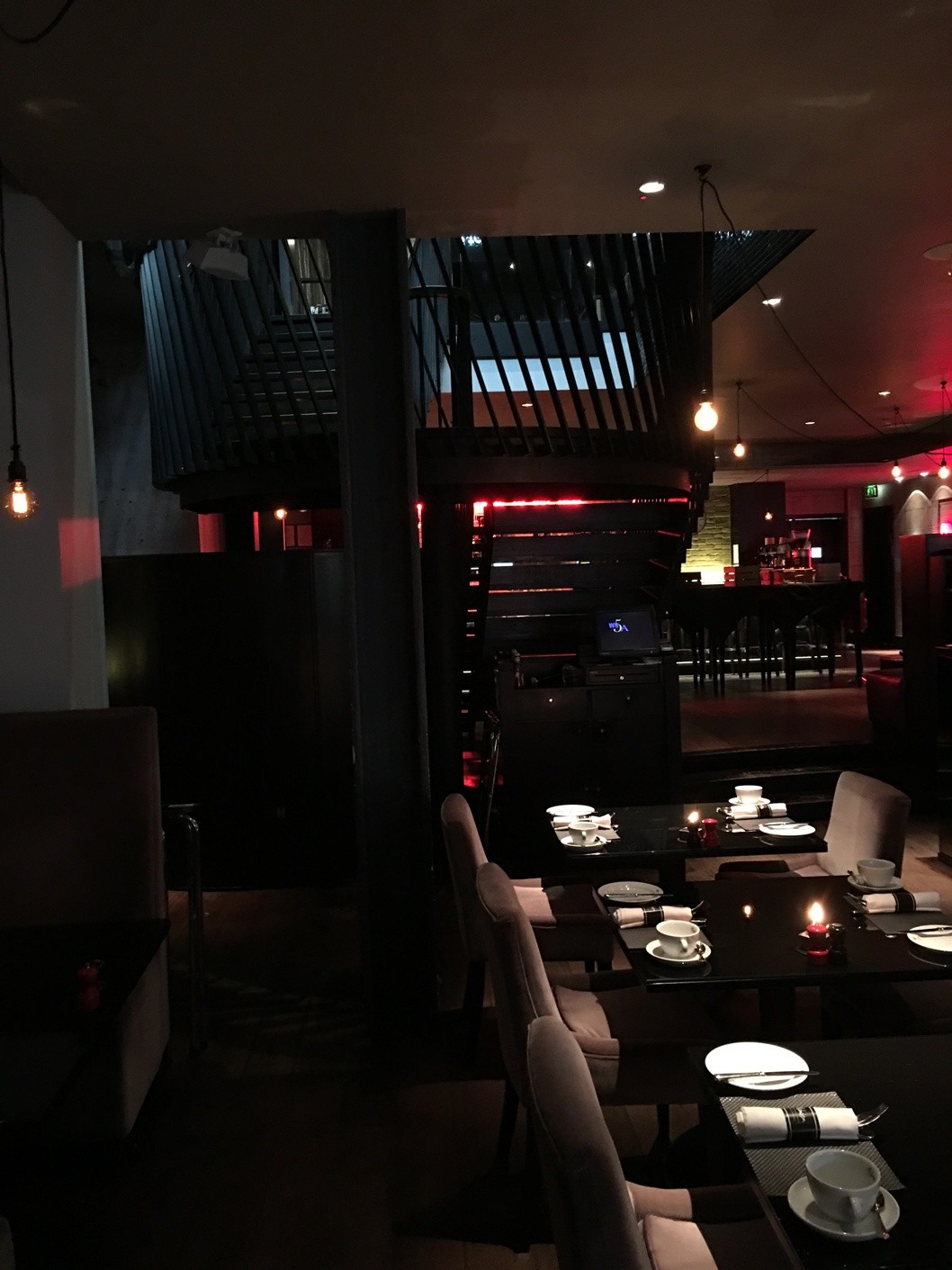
[646,940,711,965]
[788,1176,900,1242]
[546,804,595,816]
[907,924,952,953]
[705,1042,810,1091]
[560,835,607,847]
[759,821,816,836]
[598,881,664,903]
[905,938,952,967]
[728,797,771,806]
[847,874,904,893]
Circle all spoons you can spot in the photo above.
[595,836,603,843]
[695,943,707,963]
[871,1191,890,1239]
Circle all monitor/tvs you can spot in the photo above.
[594,607,662,656]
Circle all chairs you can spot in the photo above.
[719,771,911,883]
[527,1016,796,1269]
[439,794,613,1066]
[473,861,704,1195]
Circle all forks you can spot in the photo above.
[856,1102,889,1127]
[691,901,704,911]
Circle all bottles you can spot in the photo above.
[806,923,829,965]
[827,923,850,965]
[701,818,719,847]
[687,820,702,848]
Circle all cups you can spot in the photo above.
[806,1149,881,1223]
[735,785,763,803]
[656,920,700,958]
[857,859,895,888]
[568,821,599,844]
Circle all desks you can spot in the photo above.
[674,581,865,698]
[591,876,952,1043]
[1,918,170,1047]
[548,803,827,996]
[687,1035,952,1270]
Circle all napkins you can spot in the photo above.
[730,803,787,819]
[553,813,611,830]
[861,891,941,913]
[736,1106,858,1144]
[613,906,693,929]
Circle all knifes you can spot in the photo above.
[898,926,952,933]
[753,822,808,826]
[605,893,673,898]
[714,1071,820,1081]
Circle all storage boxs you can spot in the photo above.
[725,566,759,587]
[818,563,841,582]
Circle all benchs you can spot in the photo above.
[0,706,170,1184]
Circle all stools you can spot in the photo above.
[163,803,209,1051]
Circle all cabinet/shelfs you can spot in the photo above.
[498,656,680,880]
